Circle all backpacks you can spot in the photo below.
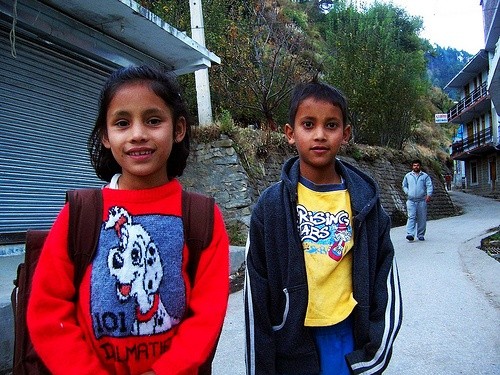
[11,187,215,375]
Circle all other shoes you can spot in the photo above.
[406,235,414,240]
[419,237,424,241]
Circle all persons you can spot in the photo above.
[26,66,230,374]
[445,173,452,190]
[403,160,433,240]
[244,81,403,375]
